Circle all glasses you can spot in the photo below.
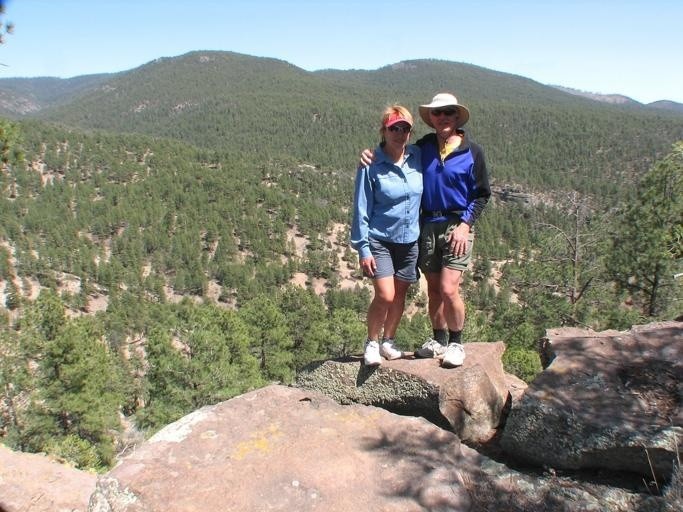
[388,125,411,134]
[431,108,456,117]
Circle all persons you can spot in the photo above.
[360,93,492,366]
[350,101,425,365]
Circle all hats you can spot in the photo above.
[417,92,470,129]
[384,114,413,129]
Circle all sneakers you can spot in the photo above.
[442,342,466,369]
[379,340,406,361]
[364,340,381,367]
[414,338,448,359]
